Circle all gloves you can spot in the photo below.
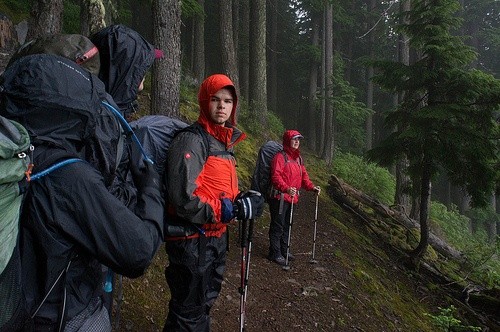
[126,151,162,195]
[234,190,261,202]
[233,195,264,220]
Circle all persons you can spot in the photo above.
[162,74,264,332]
[0,54,164,332]
[79,25,164,315]
[267,130,321,265]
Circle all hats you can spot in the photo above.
[293,134,305,140]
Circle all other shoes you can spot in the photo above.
[281,253,295,260]
[268,254,289,265]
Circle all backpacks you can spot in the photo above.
[251,140,303,204]
[0,115,86,332]
[5,31,102,84]
[127,116,210,196]
